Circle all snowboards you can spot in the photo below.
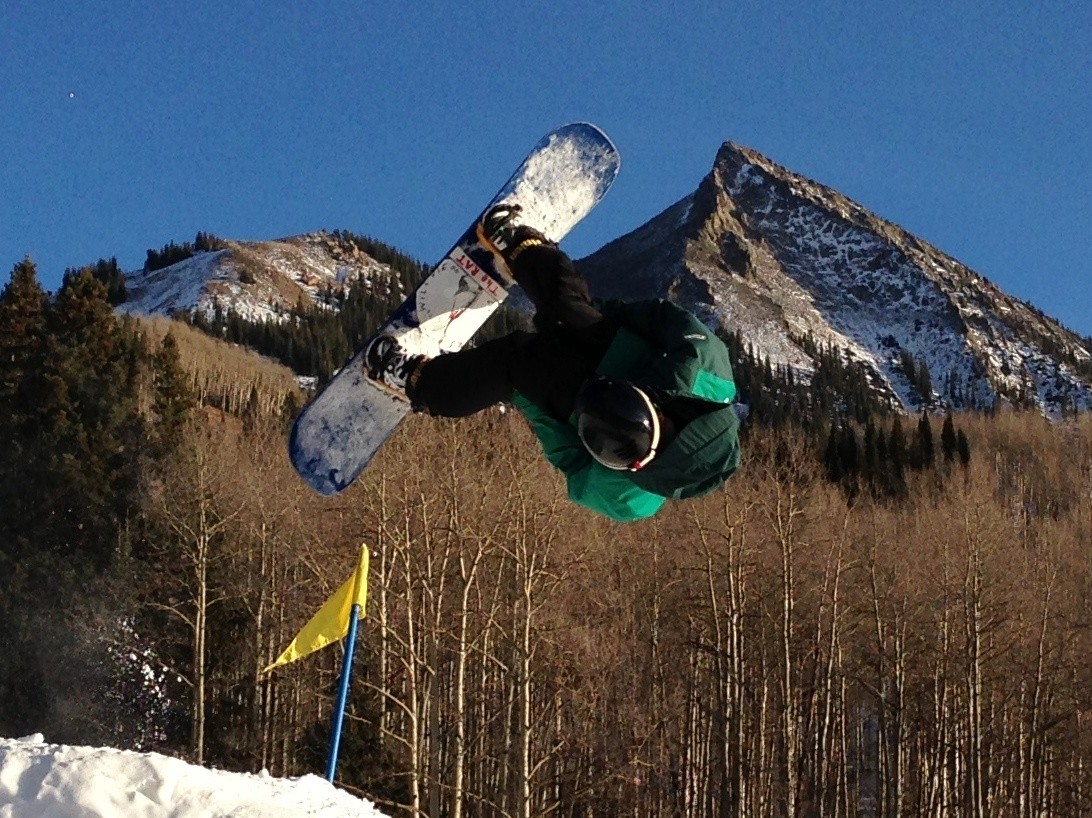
[288,122,621,496]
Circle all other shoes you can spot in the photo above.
[368,338,421,391]
[489,212,531,262]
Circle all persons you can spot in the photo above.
[366,204,743,521]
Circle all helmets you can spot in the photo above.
[577,379,653,464]
